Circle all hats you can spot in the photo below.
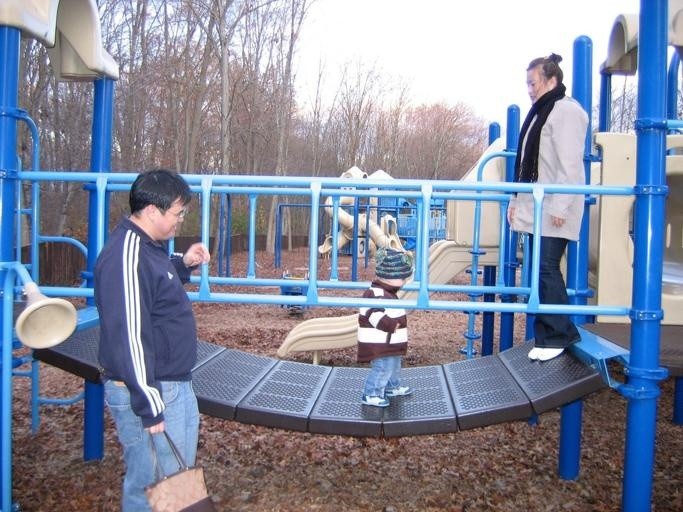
[373,245,413,279]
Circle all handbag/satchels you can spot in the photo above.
[142,431,216,512]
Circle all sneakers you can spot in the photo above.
[527,347,565,361]
[360,392,390,408]
[384,385,414,398]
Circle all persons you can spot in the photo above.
[357,246,414,407]
[94,169,210,511]
[507,53,589,363]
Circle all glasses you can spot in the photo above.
[165,206,187,219]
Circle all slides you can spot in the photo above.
[318,194,411,254]
[276,237,468,360]
